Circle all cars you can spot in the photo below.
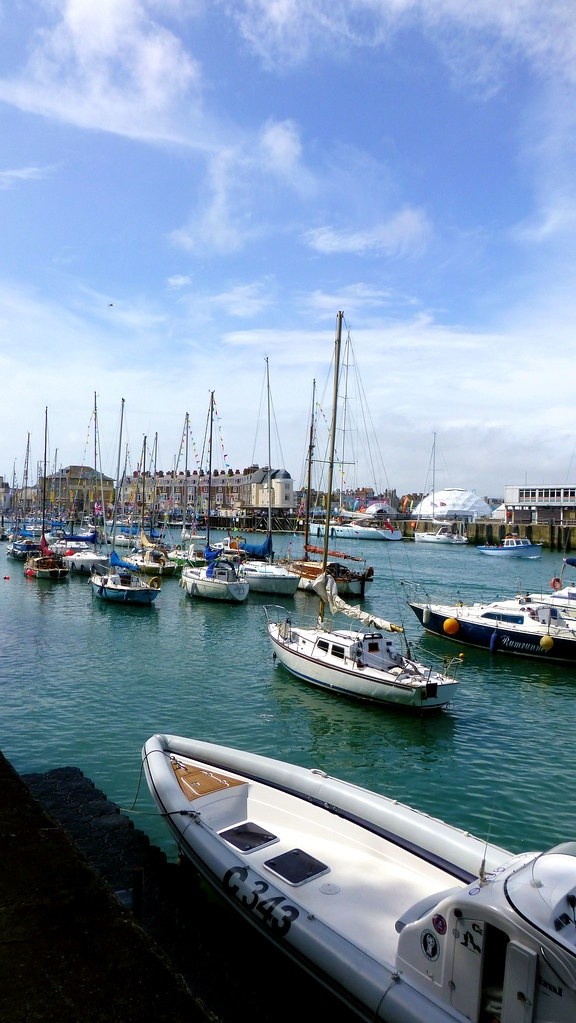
[302,506,327,518]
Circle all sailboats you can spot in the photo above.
[232,356,301,595]
[122,436,184,576]
[309,330,402,541]
[89,398,162,603]
[7,432,113,560]
[274,378,375,595]
[264,310,463,709]
[24,407,70,579]
[167,412,227,567]
[415,433,470,544]
[181,389,250,601]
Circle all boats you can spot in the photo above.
[142,733,576,1023]
[476,536,543,559]
[401,558,576,664]
[212,537,248,556]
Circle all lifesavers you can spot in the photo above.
[551,577,561,590]
[149,577,162,589]
